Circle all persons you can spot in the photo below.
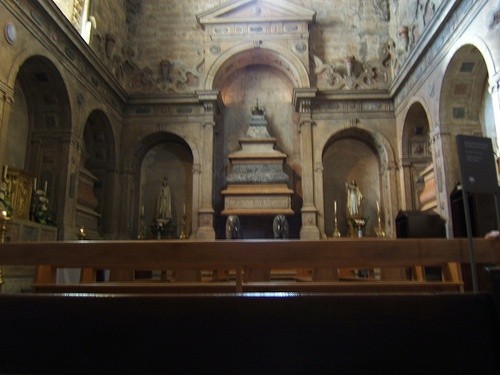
[157,179,172,220]
[345,180,366,218]
